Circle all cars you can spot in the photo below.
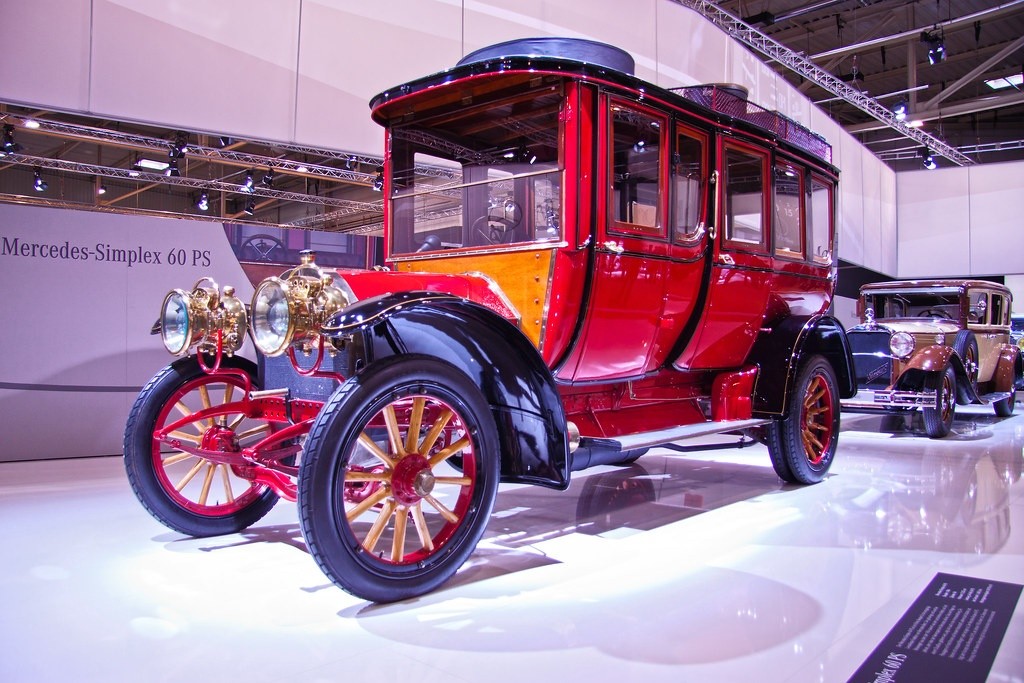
[839,279,1024,438]
[123,37,858,604]
